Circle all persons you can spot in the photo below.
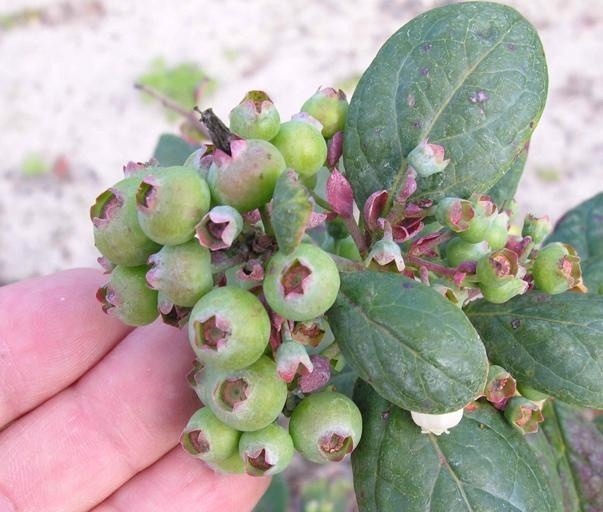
[0,266,273,510]
